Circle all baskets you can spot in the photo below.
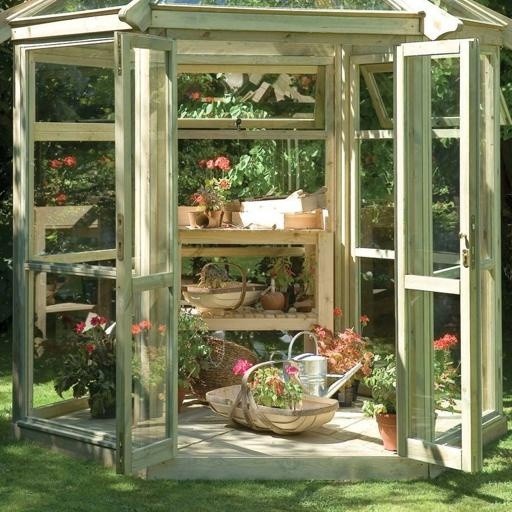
[187,336,261,404]
[206,360,340,436]
[183,263,268,309]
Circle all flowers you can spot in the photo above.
[233,358,303,408]
[193,154,230,207]
[47,153,78,206]
[309,305,374,386]
[53,305,210,419]
[435,333,460,409]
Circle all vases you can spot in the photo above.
[187,211,224,228]
[336,386,354,407]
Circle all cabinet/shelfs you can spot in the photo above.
[178,226,335,353]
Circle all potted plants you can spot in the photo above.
[362,354,399,453]
